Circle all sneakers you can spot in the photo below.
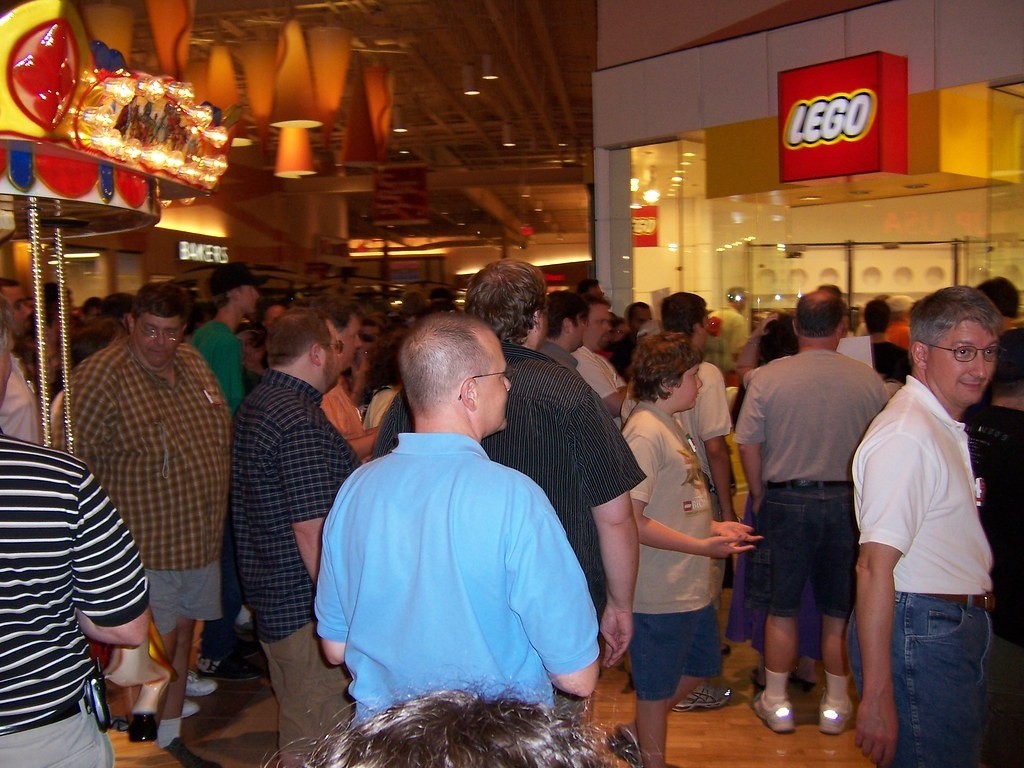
[183,670,218,695]
[754,689,795,732]
[818,694,855,733]
[182,698,200,718]
[672,686,733,711]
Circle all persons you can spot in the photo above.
[846,286,1003,768]
[263,690,638,766]
[0,296,152,768]
[0,256,1024,735]
[313,312,600,727]
[965,327,1024,768]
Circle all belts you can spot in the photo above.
[932,594,995,612]
[767,479,843,490]
[1,697,90,737]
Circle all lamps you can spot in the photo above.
[79,0,395,180]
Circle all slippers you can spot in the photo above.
[789,671,813,691]
[750,667,765,688]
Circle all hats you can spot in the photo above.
[661,292,707,331]
[402,291,423,313]
[210,262,270,295]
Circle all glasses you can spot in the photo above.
[457,364,518,400]
[613,330,625,336]
[320,340,345,355]
[926,346,1007,361]
[137,320,181,344]
